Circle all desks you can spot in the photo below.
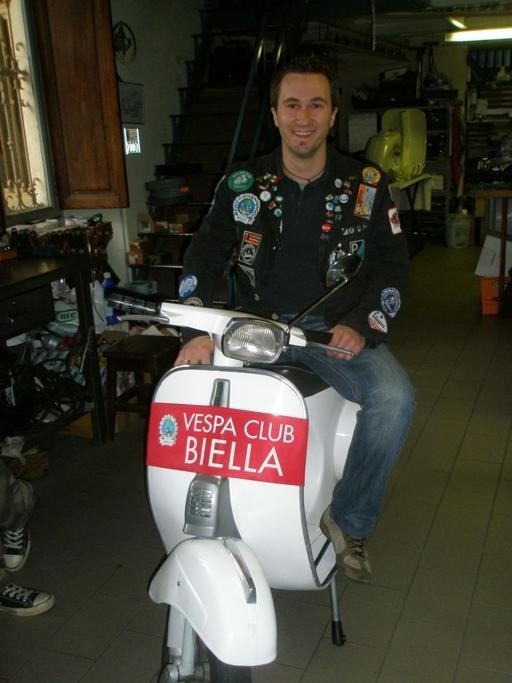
[0,256,107,449]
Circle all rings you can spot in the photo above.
[182,356,189,362]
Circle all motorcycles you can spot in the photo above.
[101,287,355,682]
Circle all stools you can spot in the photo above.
[103,333,182,442]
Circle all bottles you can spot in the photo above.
[35,332,69,351]
[101,271,120,325]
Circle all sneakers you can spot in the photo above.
[318,503,372,584]
[0,582,58,618]
[3,479,33,573]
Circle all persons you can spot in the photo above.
[1,454,57,622]
[171,47,419,582]
